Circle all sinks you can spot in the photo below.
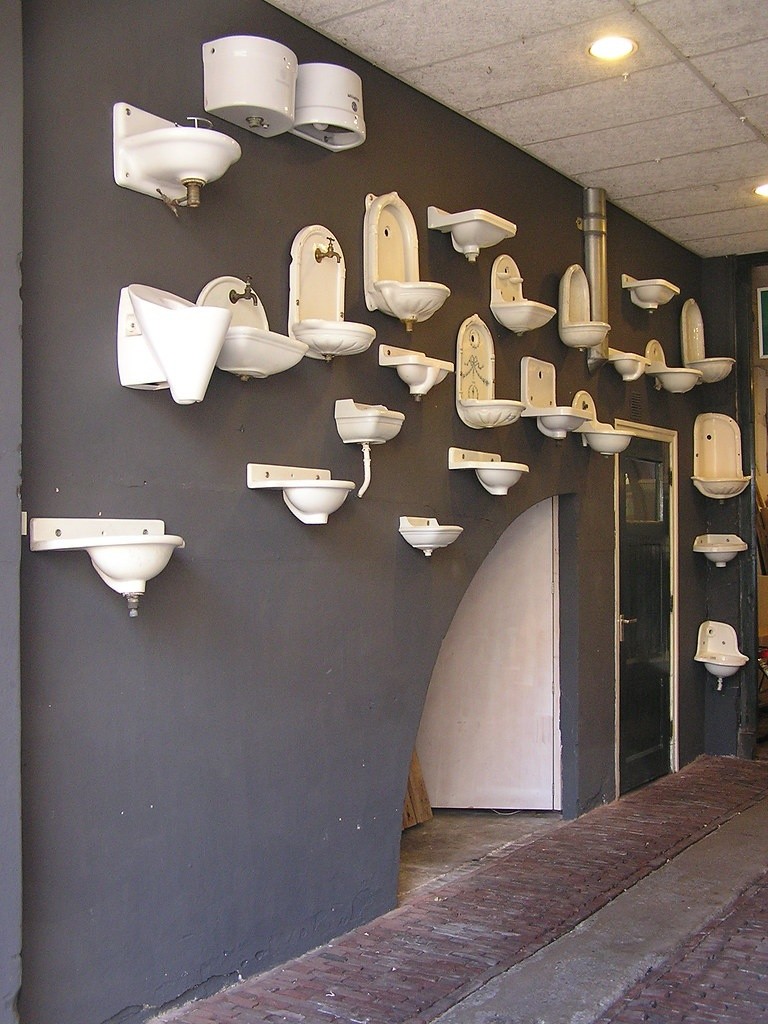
[693,534,748,568]
[112,100,243,218]
[288,191,752,499]
[693,620,749,691]
[246,463,356,525]
[397,514,464,557]
[118,275,312,406]
[28,518,187,618]
[202,35,367,153]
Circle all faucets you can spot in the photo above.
[229,275,258,307]
[314,236,341,264]
